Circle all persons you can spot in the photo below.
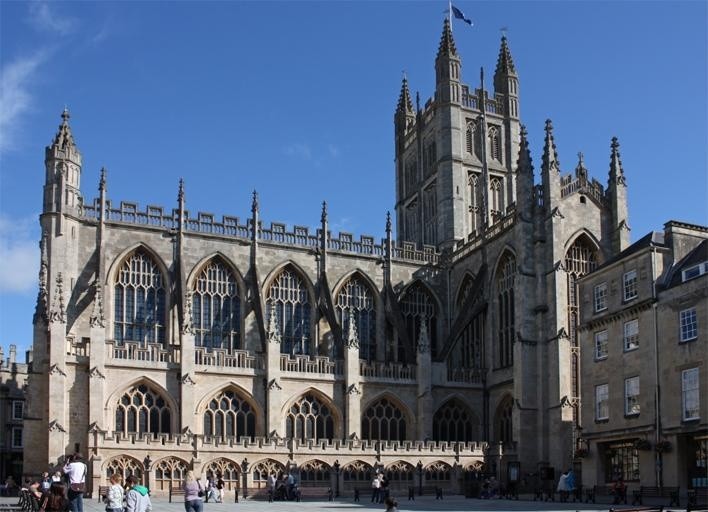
[381,475,390,503]
[196,477,204,497]
[369,471,383,504]
[216,475,225,503]
[612,477,626,505]
[487,476,500,499]
[205,476,211,502]
[556,472,571,503]
[181,472,204,512]
[103,473,125,511]
[207,474,218,502]
[384,496,399,512]
[481,478,495,499]
[122,474,152,511]
[377,471,386,504]
[122,476,130,501]
[267,469,304,503]
[0,452,88,512]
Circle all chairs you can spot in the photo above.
[18,487,40,512]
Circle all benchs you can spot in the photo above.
[593,485,627,505]
[632,486,680,507]
[236,487,331,504]
[169,486,185,503]
[354,486,389,502]
[687,489,708,512]
[408,486,443,500]
[99,485,109,502]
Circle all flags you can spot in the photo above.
[450,4,474,28]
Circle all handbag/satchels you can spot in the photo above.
[69,483,87,493]
[198,491,205,497]
